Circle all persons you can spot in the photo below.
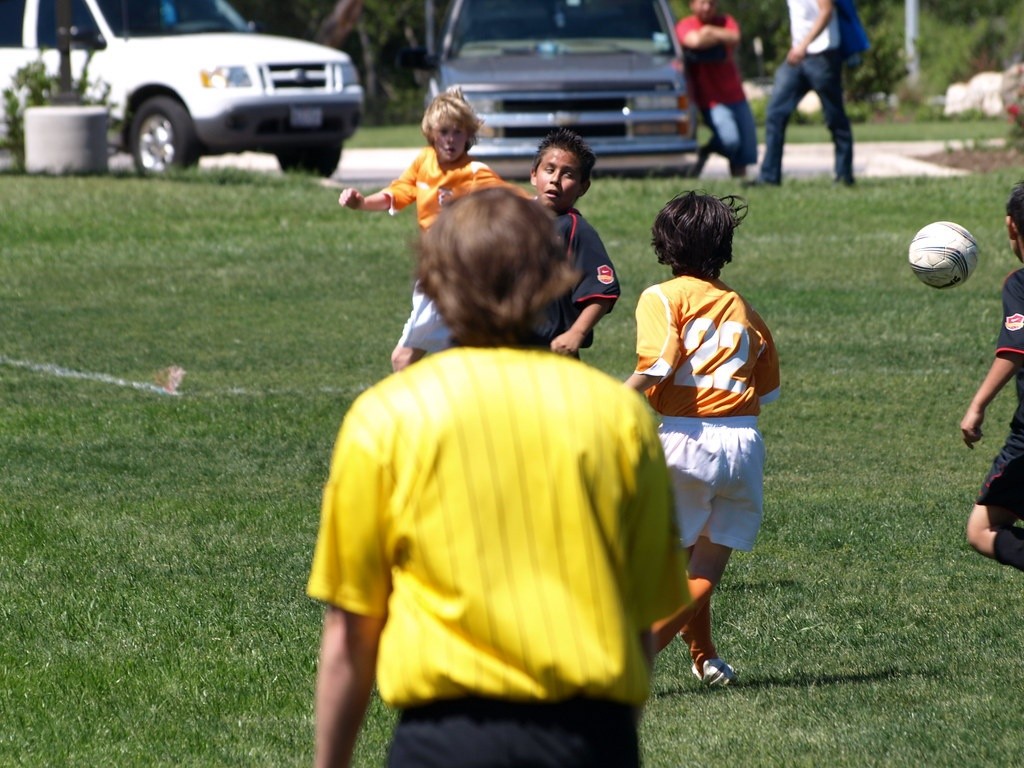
[531,129,621,361]
[338,90,532,372]
[961,181,1024,575]
[675,0,757,177]
[306,189,691,767]
[744,0,855,187]
[624,191,780,686]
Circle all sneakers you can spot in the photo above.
[692,658,735,687]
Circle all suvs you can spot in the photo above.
[0,0,365,179]
[396,0,727,180]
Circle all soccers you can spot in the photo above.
[908,221,979,290]
